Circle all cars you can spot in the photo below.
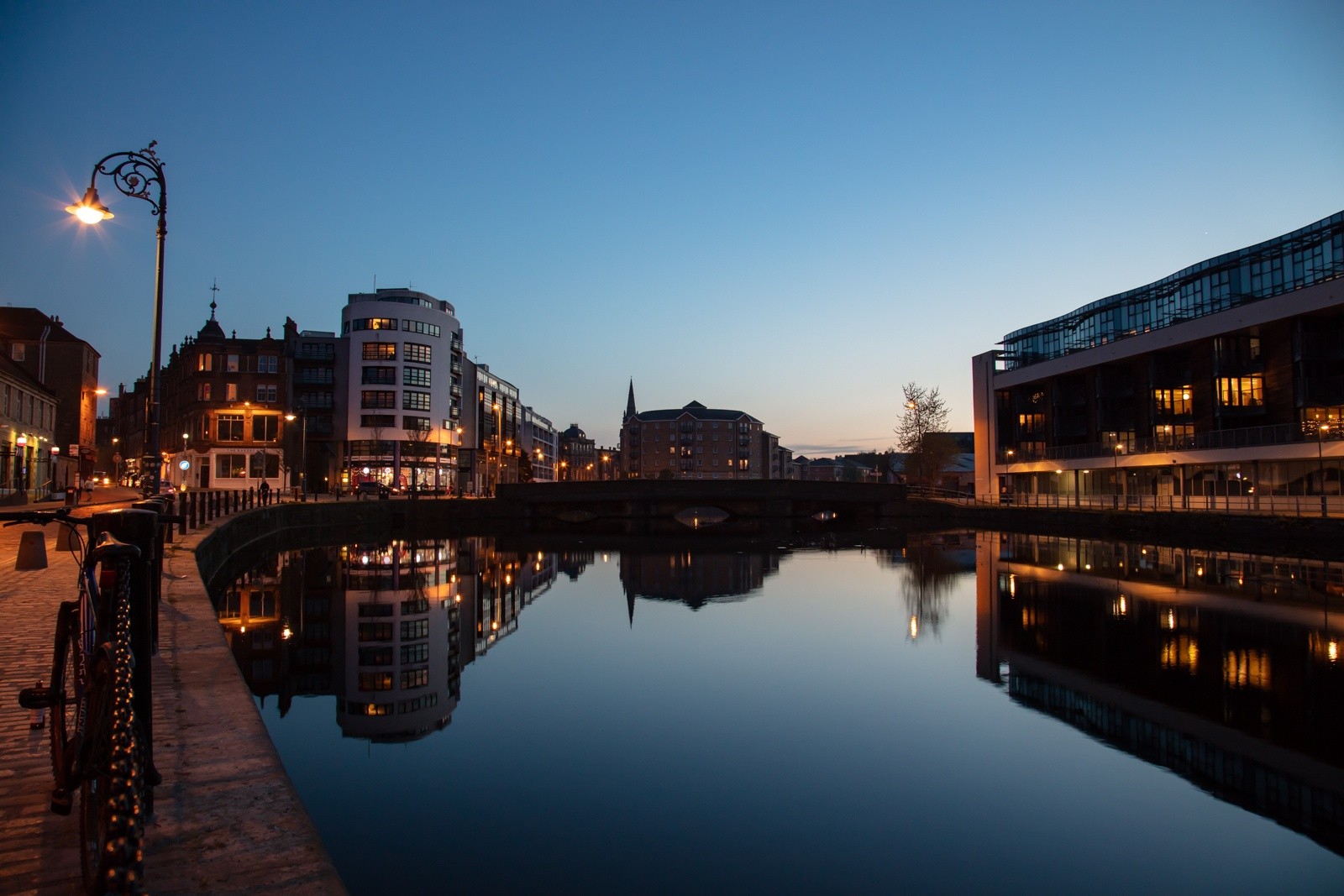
[356,482,400,495]
[118,472,149,488]
[159,479,175,498]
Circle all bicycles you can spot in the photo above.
[0,507,186,896]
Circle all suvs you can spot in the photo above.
[94,472,108,485]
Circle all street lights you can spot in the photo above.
[1006,449,1013,505]
[1113,546,1126,622]
[284,408,308,493]
[907,402,921,487]
[112,437,119,487]
[449,538,461,602]
[245,401,268,483]
[283,549,302,645]
[1115,444,1123,508]
[491,563,501,631]
[603,455,608,480]
[456,427,462,496]
[1324,559,1338,663]
[494,404,501,484]
[1318,424,1330,517]
[182,433,189,485]
[65,140,169,506]
[1007,533,1015,599]
